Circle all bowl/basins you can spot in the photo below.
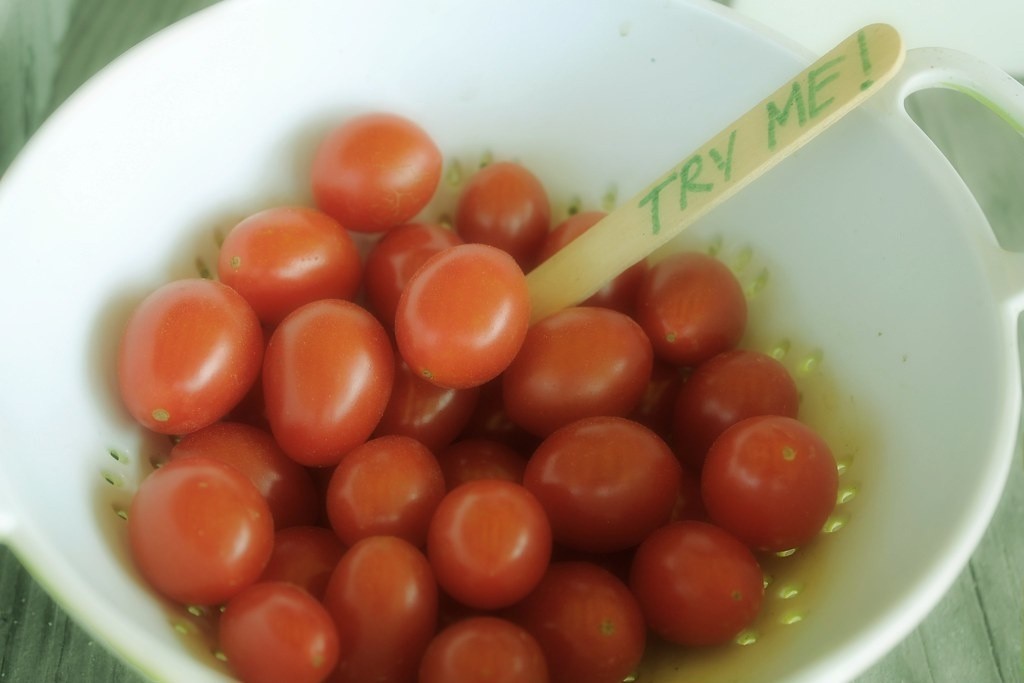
[0,0,1024,683]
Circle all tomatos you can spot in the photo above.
[120,115,839,683]
